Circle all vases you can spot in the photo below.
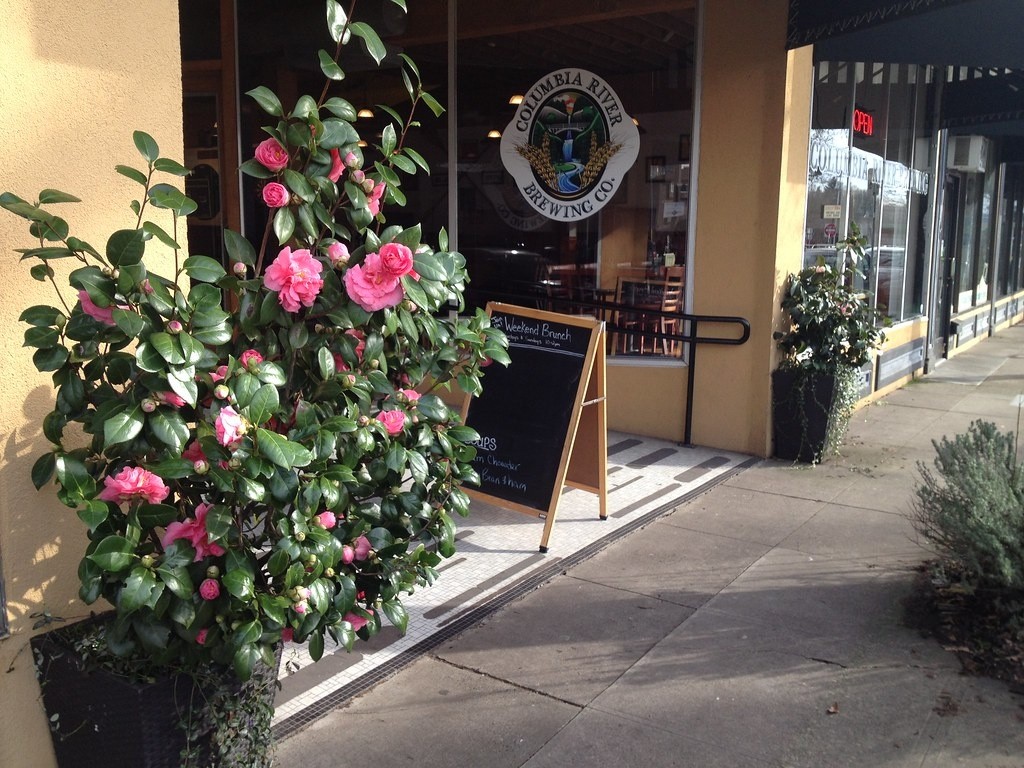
[28,609,285,768]
[773,367,859,465]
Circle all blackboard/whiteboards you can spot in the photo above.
[440,303,599,521]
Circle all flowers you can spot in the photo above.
[0,0,512,674]
[772,219,897,363]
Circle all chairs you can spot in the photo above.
[504,255,685,357]
